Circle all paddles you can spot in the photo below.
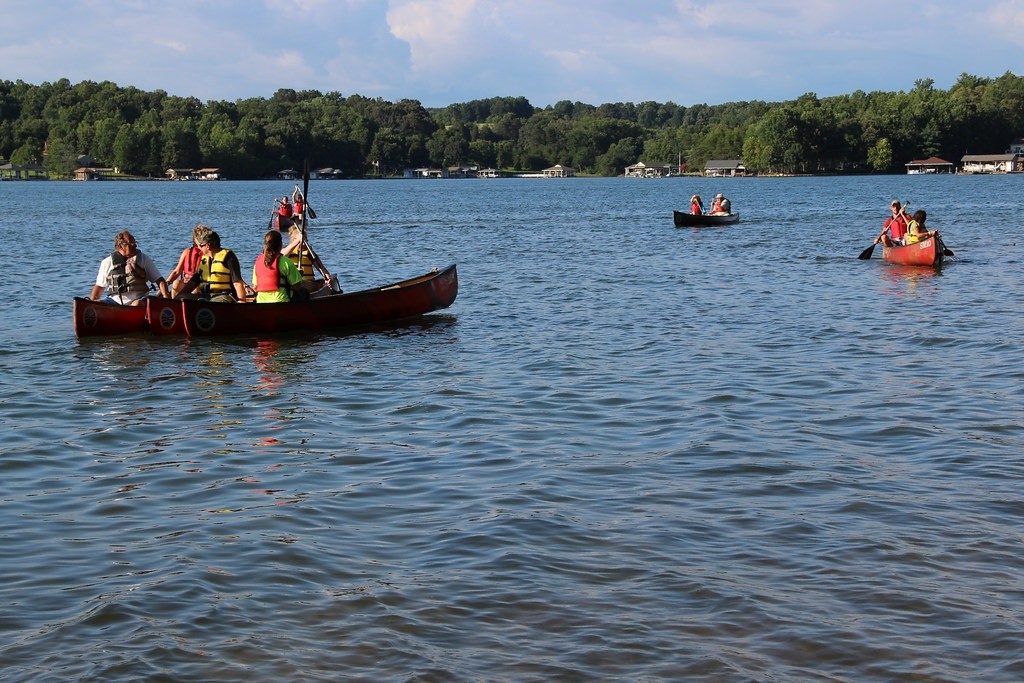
[858,200,911,261]
[295,184,316,219]
[123,284,157,305]
[708,198,714,215]
[290,215,337,294]
[296,157,310,269]
[268,199,277,231]
[935,231,954,257]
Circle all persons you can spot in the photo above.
[874,201,912,247]
[708,193,731,216]
[293,187,309,219]
[280,225,335,297]
[91,231,170,306]
[907,210,942,245]
[251,230,303,303]
[690,195,706,216]
[274,196,292,218]
[173,231,247,303]
[156,225,212,299]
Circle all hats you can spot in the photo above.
[715,193,724,198]
[690,195,700,202]
[891,200,900,206]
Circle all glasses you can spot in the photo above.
[122,241,138,246]
[199,243,206,247]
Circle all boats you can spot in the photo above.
[672,208,740,228]
[272,216,309,231]
[884,237,944,264]
[71,263,459,343]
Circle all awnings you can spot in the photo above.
[277,168,299,180]
